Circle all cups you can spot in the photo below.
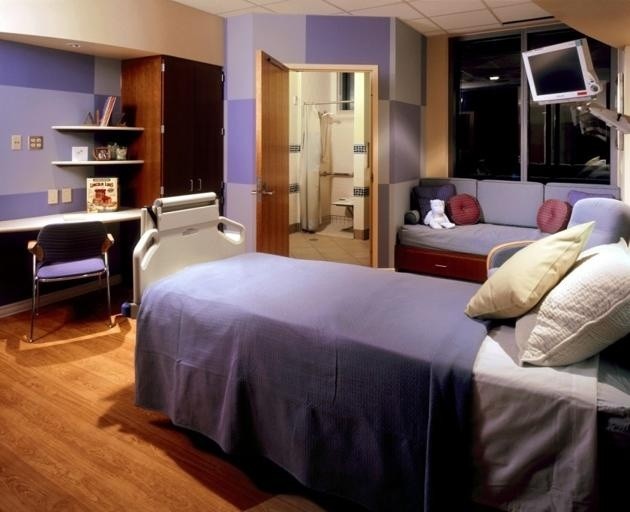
[92,147,111,160]
[115,147,128,160]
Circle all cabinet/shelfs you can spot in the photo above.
[120,54,225,234]
[0,126,145,233]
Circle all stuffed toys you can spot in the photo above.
[423,198,456,230]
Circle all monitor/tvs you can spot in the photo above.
[520,38,601,102]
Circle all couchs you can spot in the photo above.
[394,178,621,284]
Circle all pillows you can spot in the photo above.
[536,191,614,234]
[411,184,481,225]
[464,219,629,369]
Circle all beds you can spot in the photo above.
[130,191,630,511]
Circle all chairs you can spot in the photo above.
[486,197,630,285]
[26,220,116,343]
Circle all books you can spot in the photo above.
[100,95,118,126]
[86,176,119,214]
